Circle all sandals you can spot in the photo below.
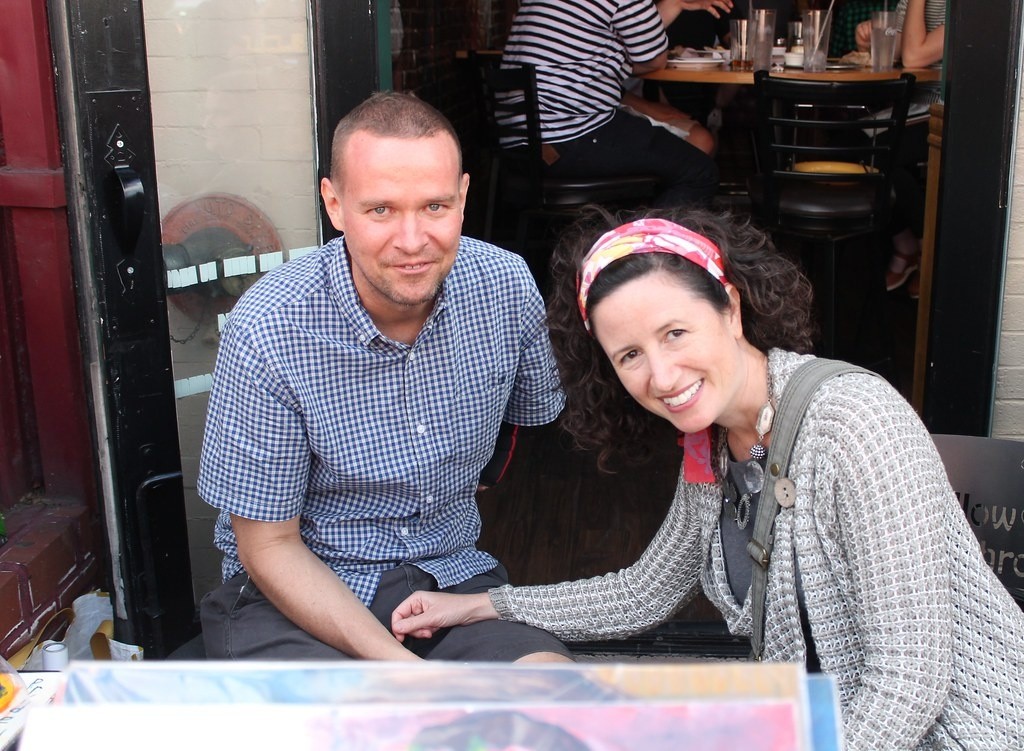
[907,265,925,298]
[879,239,920,293]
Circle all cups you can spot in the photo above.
[870,11,897,75]
[802,10,832,72]
[786,20,807,69]
[748,9,776,71]
[730,19,755,71]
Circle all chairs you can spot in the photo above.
[465,47,659,294]
[751,69,916,342]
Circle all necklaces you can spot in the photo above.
[716,374,774,531]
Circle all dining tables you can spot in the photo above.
[639,46,938,87]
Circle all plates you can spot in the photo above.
[676,49,734,61]
[666,57,725,70]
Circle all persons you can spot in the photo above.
[856,0,945,298]
[196,89,569,667]
[392,203,1022,751]
[618,78,717,156]
[494,1,733,203]
